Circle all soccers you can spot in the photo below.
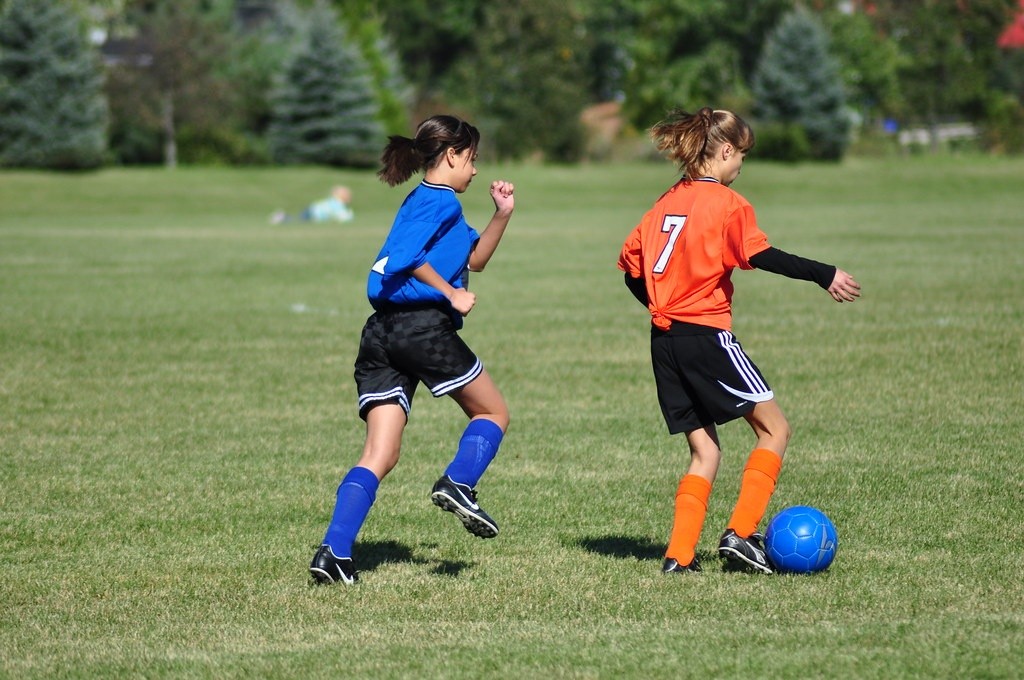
[763,505,837,573]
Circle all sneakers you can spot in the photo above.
[431,476,499,539]
[719,528,775,576]
[662,557,702,574]
[310,544,358,585]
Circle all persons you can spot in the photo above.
[615,109,861,576]
[307,116,515,587]
[267,185,354,227]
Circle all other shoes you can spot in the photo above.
[272,213,284,221]
